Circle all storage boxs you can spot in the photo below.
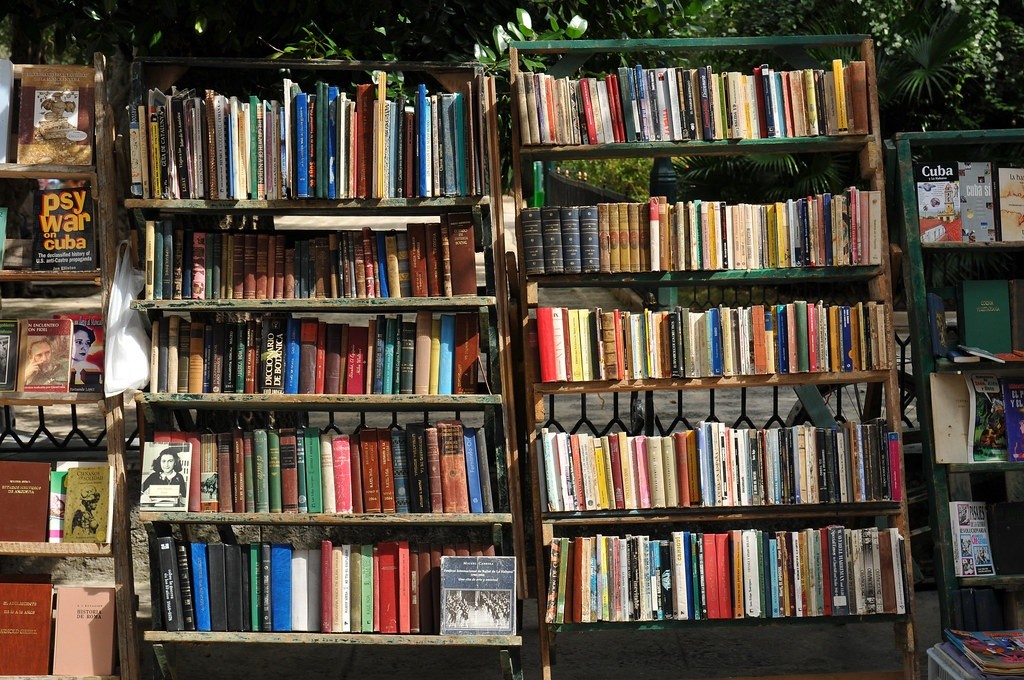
[926,642,975,680]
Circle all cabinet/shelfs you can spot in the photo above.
[0,33,138,680]
[883,128,1024,642]
[124,55,527,680]
[509,34,917,679]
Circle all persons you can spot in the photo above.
[143,449,186,498]
[25,337,68,384]
[71,325,101,381]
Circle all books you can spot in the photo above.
[129,71,516,635]
[516,59,906,625]
[944,628,1024,676]
[915,159,1024,578]
[0,57,115,676]
